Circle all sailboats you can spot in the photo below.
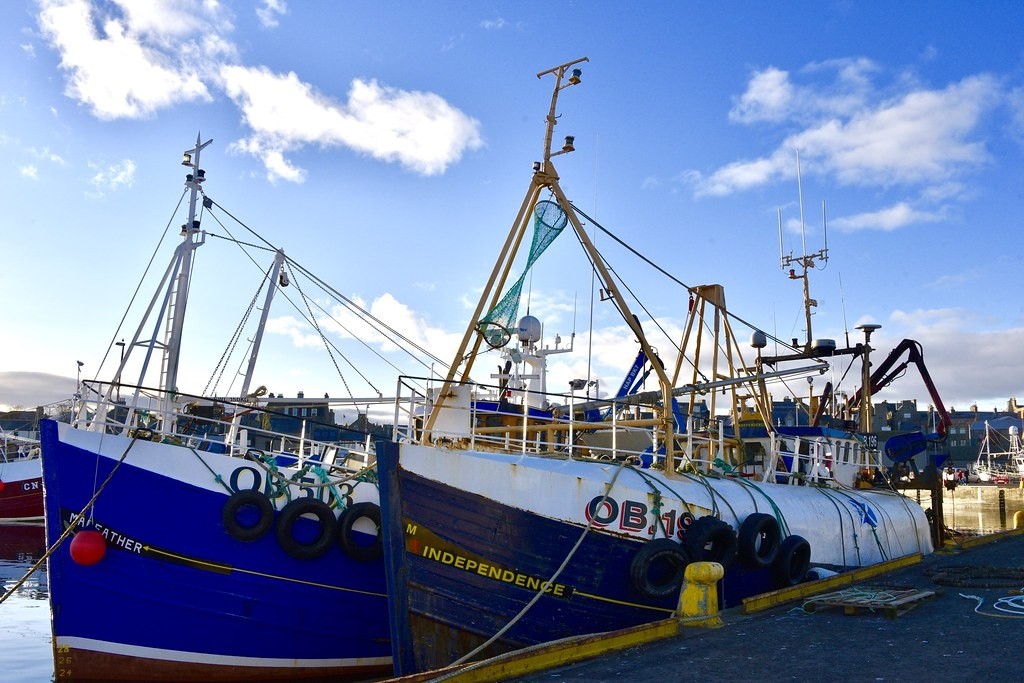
[972,418,1023,483]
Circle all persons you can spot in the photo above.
[958,471,962,481]
[964,468,969,484]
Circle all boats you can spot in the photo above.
[29,129,478,683]
[375,53,952,683]
[0,424,47,562]
